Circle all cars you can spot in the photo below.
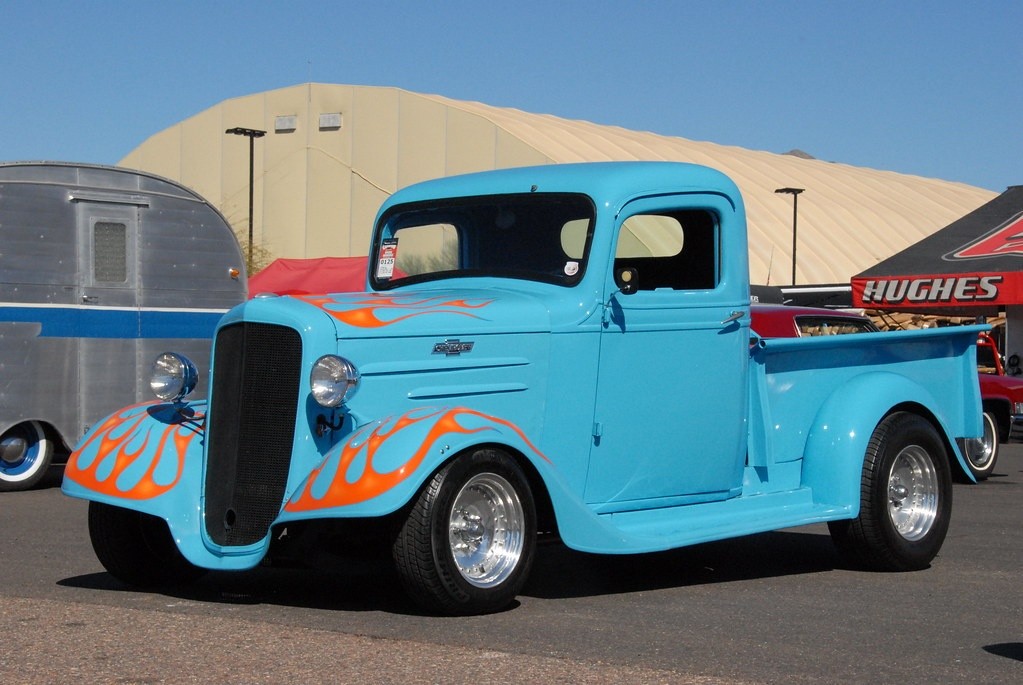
[750,303,1023,481]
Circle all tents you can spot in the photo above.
[851,186,1023,443]
[244,255,411,295]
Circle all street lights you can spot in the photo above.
[225,127,267,278]
[775,187,806,288]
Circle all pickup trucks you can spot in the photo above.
[57,155,997,617]
